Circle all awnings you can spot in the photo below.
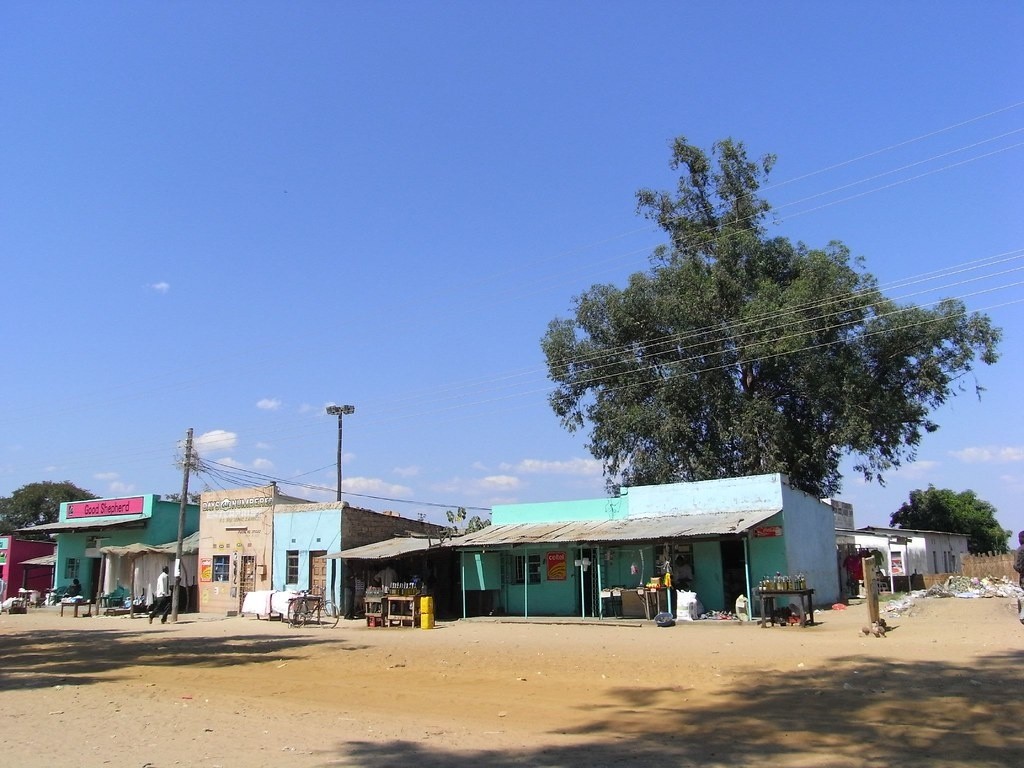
[319,537,451,562]
[13,516,150,532]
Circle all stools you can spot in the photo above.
[36,598,47,608]
[101,597,109,608]
[12,600,22,607]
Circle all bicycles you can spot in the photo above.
[288,584,340,629]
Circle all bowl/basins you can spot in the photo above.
[655,614,673,626]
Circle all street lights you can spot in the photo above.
[326,405,355,501]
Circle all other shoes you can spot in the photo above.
[149,616,152,624]
[162,621,166,623]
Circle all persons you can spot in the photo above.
[1013,531,1024,590]
[149,566,172,624]
[374,563,398,589]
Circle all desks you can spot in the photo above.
[60,602,92,617]
[240,590,322,623]
[757,588,816,629]
[17,591,42,608]
[364,594,427,628]
[598,586,669,620]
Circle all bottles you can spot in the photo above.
[390,581,417,597]
[759,571,806,591]
[421,583,425,595]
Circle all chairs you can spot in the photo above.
[109,593,124,607]
